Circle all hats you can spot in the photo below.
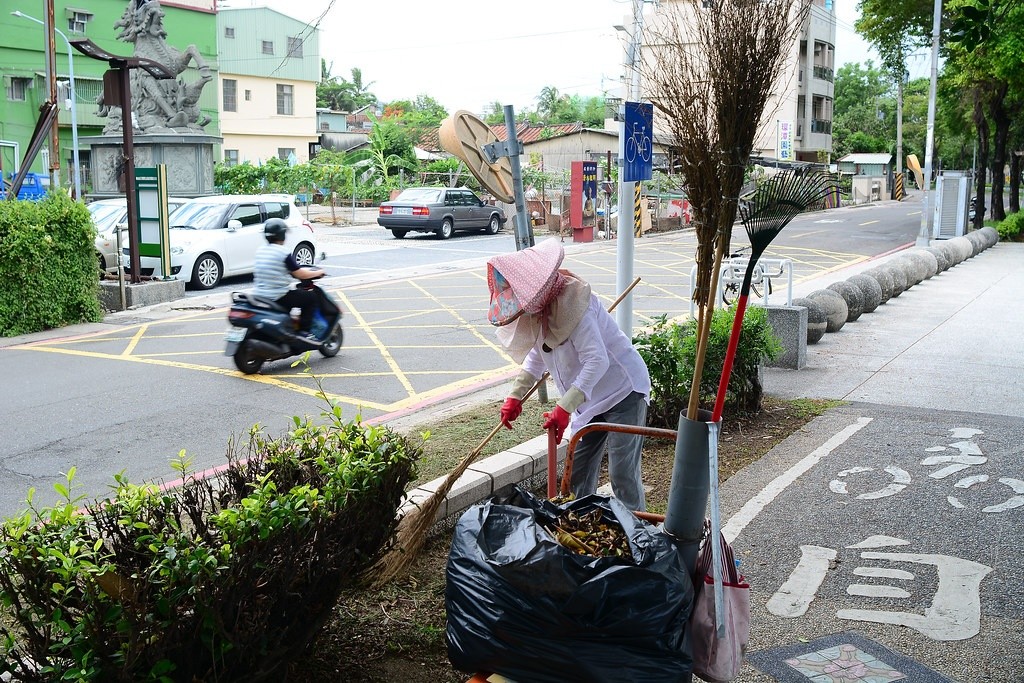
[488,238,565,328]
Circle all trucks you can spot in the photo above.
[0,170,50,203]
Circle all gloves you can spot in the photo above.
[543,386,585,445]
[501,368,537,430]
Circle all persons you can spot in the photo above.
[252,217,327,346]
[486,237,653,522]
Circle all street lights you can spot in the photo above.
[613,24,641,343]
[8,9,82,198]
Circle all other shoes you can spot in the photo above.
[298,330,316,339]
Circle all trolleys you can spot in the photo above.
[562,422,675,522]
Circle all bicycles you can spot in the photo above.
[721,245,772,305]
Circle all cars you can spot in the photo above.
[118,193,317,291]
[375,185,508,239]
[77,197,196,284]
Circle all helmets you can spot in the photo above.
[265,218,290,235]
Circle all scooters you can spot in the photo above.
[967,195,987,222]
[223,251,344,376]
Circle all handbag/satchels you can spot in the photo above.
[309,308,330,339]
[679,519,750,682]
